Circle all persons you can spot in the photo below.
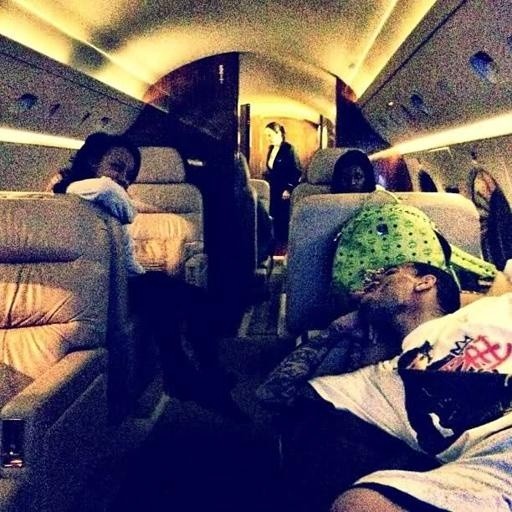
[260,121,302,243]
[248,257,512,512]
[52,127,241,419]
[328,148,386,193]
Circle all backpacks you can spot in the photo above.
[329,189,498,321]
